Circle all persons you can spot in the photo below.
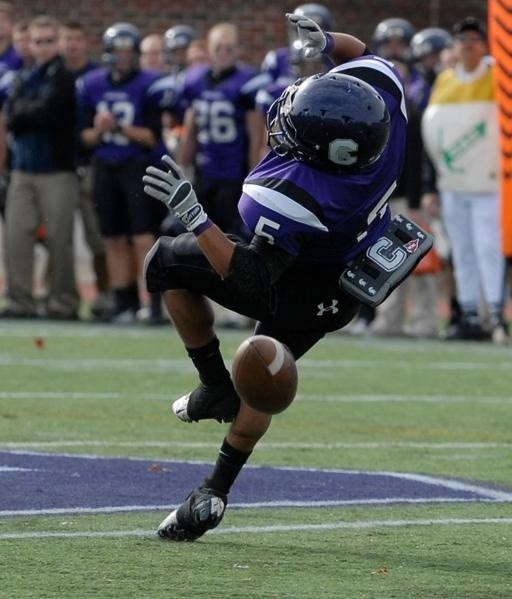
[143,11,406,542]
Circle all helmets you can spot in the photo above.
[293,2,331,30]
[161,25,197,64]
[97,23,141,68]
[372,15,455,67]
[263,68,394,177]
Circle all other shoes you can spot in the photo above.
[0,301,170,324]
[349,318,507,342]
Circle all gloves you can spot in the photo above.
[141,149,210,238]
[283,7,328,61]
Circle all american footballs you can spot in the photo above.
[231,335,298,415]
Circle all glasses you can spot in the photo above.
[29,34,60,48]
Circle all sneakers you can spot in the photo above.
[168,385,243,425]
[151,492,230,542]
